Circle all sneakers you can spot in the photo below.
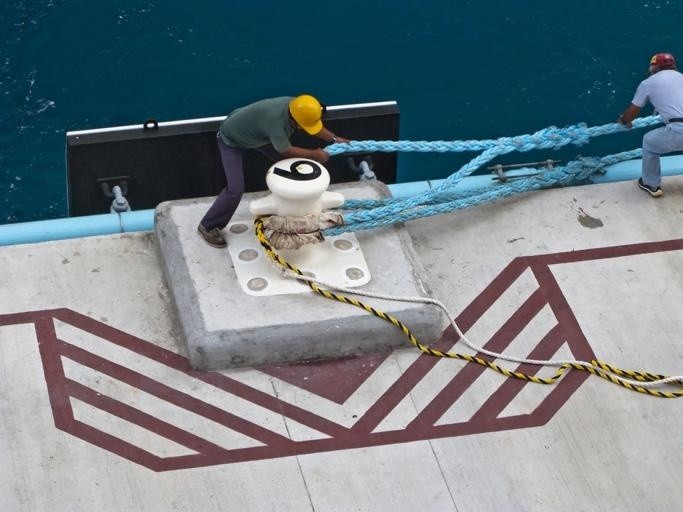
[197,221,226,248]
[638,177,664,196]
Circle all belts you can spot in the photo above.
[669,117,683,123]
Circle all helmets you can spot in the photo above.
[289,94,323,135]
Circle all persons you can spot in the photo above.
[618,52,683,197]
[194,93,351,250]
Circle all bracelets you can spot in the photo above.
[329,134,338,144]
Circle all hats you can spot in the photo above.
[647,53,675,76]
